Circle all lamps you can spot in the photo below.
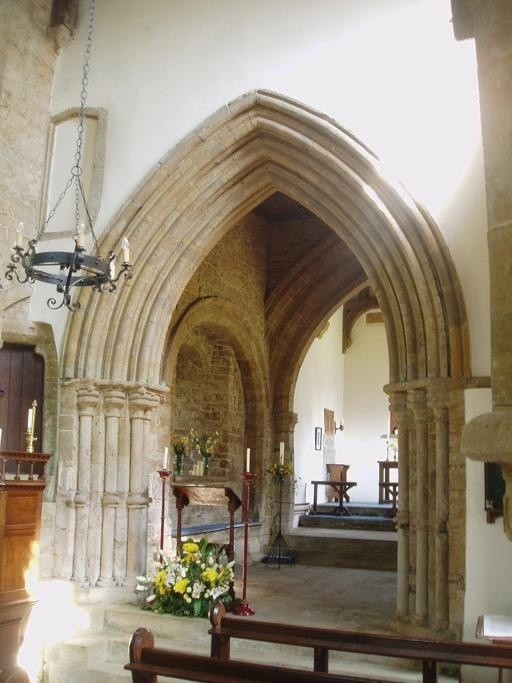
[2,1,135,313]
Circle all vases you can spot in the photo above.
[174,453,185,477]
[203,455,212,476]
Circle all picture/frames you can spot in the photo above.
[315,427,323,451]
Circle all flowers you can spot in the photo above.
[133,538,237,618]
[171,425,221,459]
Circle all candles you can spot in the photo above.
[163,446,169,469]
[246,447,251,472]
[279,441,285,464]
[28,408,33,428]
